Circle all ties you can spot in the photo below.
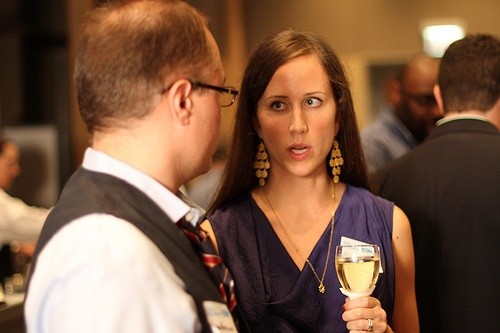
[174,215,240,333]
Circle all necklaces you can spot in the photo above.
[261,179,334,294]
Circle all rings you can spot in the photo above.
[367,319,374,331]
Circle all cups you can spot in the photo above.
[335,244,382,301]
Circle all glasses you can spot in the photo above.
[160,79,238,108]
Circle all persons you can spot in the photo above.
[366,32,500,333]
[200,30,420,333]
[0,134,55,288]
[360,54,442,174]
[21,1,240,333]
